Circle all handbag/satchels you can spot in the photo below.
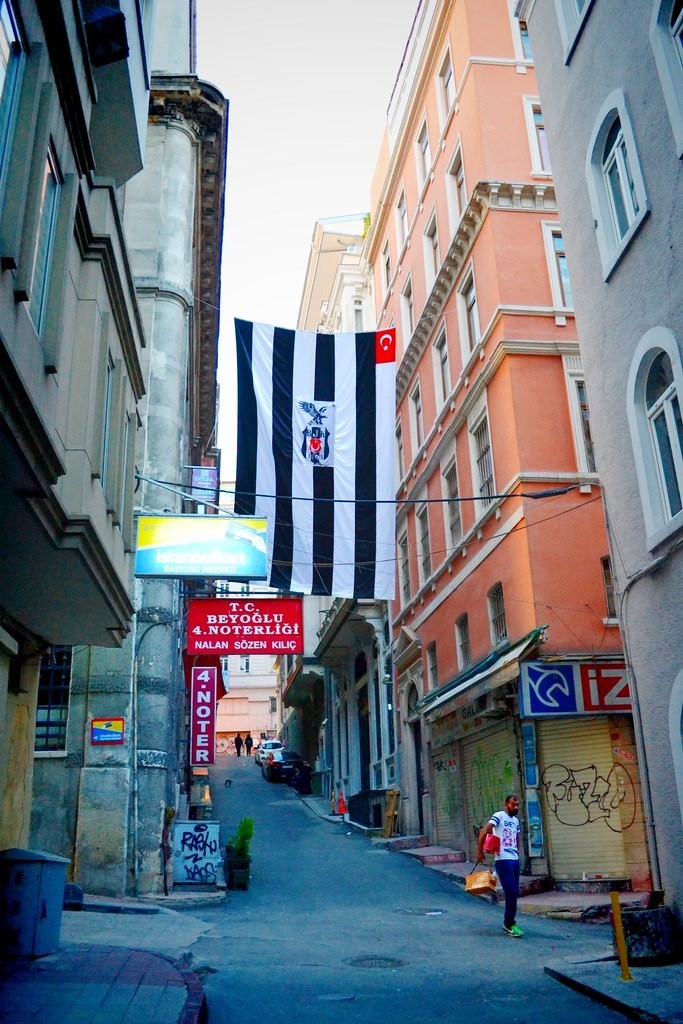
[465,862,497,895]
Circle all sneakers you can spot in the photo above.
[502,924,524,937]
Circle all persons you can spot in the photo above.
[295,761,314,794]
[235,733,244,757]
[477,795,524,936]
[244,734,252,755]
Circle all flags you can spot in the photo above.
[234,316,397,602]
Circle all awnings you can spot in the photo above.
[421,632,539,724]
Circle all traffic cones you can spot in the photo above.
[335,790,347,815]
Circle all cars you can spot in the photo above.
[261,750,302,783]
[253,739,285,767]
